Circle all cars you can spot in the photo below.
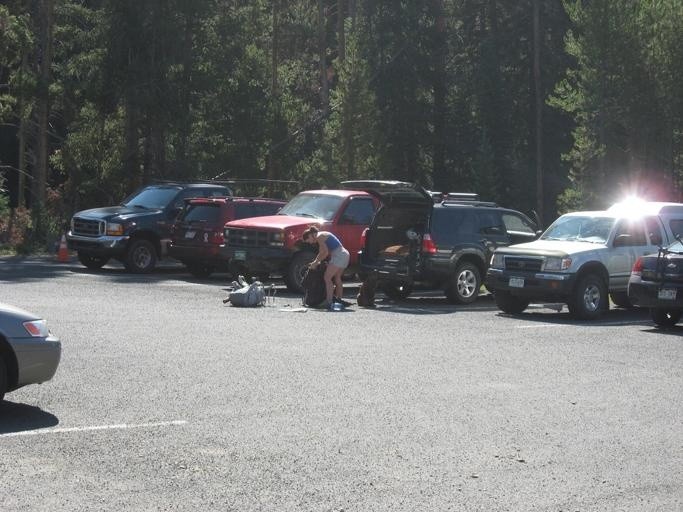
[0,303,60,403]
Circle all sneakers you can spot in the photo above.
[315,299,331,309]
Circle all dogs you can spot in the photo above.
[356,269,380,307]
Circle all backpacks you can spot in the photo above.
[228,274,277,307]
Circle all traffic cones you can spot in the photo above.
[58,233,69,262]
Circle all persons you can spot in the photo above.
[300,224,351,309]
